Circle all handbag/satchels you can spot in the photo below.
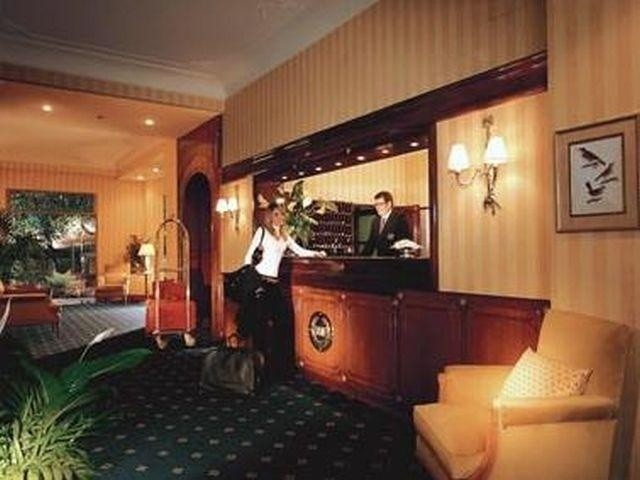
[220,263,262,301]
[196,346,273,397]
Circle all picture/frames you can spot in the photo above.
[554,112,640,233]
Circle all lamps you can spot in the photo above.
[447,115,509,217]
[215,183,240,234]
[138,243,156,275]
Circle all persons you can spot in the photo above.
[241,201,328,387]
[358,190,414,258]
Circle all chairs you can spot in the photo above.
[94,272,130,306]
[0,278,60,339]
[412,306,640,479]
[129,274,154,305]
[146,276,200,350]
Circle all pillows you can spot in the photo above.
[498,344,593,399]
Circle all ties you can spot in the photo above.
[378,219,386,235]
